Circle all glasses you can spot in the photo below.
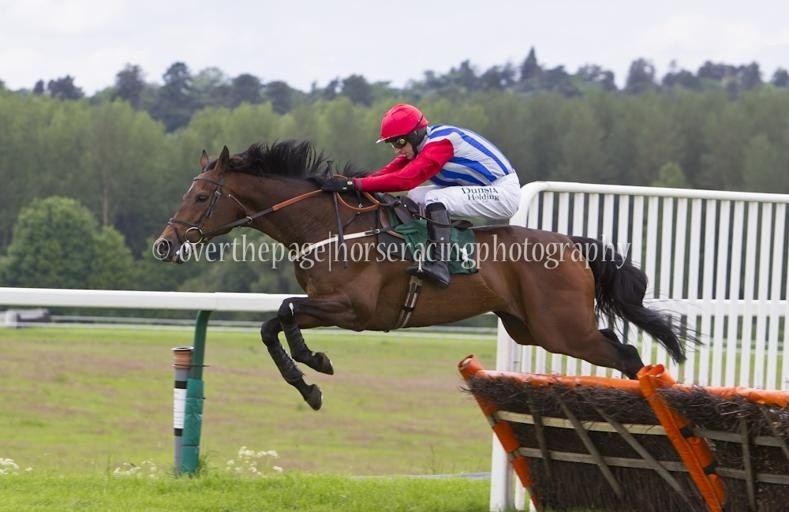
[392,137,407,148]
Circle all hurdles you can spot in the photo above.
[458,353,789,512]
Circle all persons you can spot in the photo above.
[322,103,521,287]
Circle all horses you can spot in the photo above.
[151,136,707,412]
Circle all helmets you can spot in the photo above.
[375,104,428,144]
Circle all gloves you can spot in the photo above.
[320,175,353,192]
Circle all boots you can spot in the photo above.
[403,210,453,289]
